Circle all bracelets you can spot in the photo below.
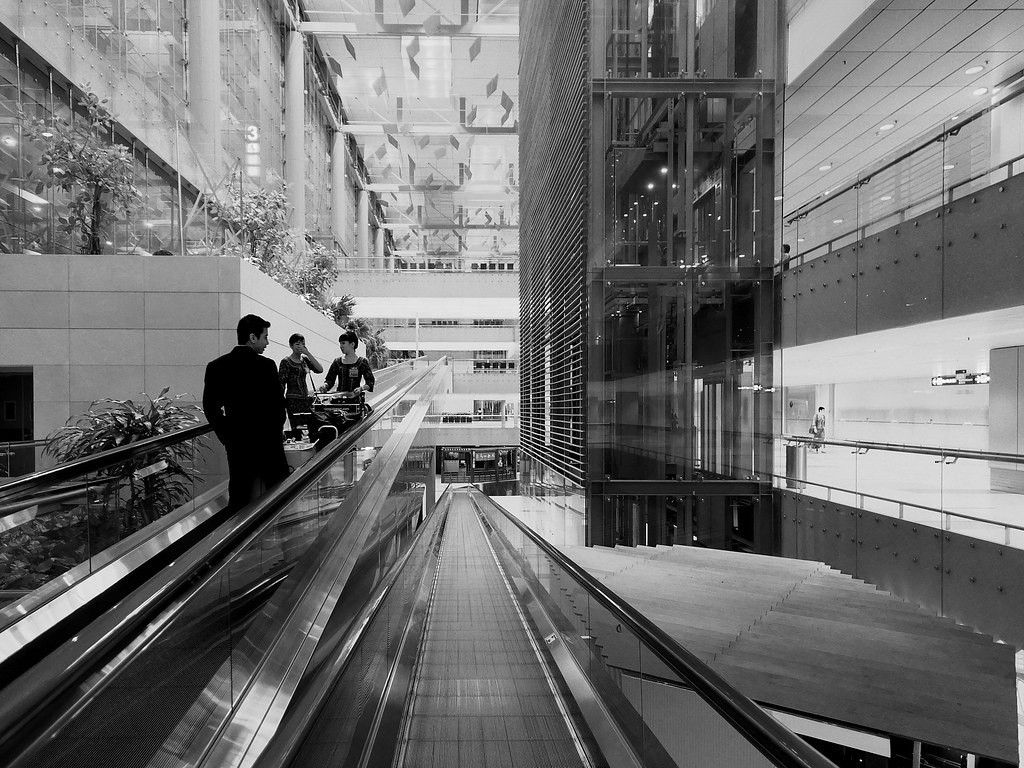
[361,385,365,390]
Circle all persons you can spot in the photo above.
[498,457,503,467]
[279,332,329,450]
[812,407,827,453]
[318,331,375,396]
[779,244,790,271]
[478,407,482,420]
[202,314,291,517]
[402,350,424,359]
